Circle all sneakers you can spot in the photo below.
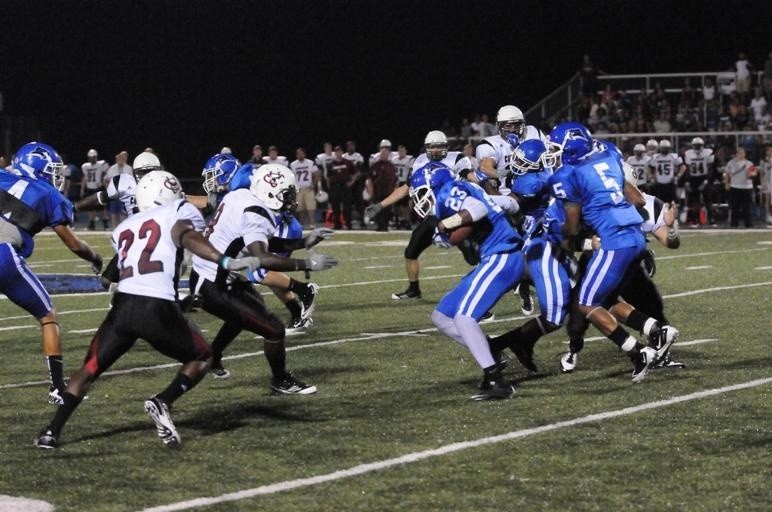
[285,315,313,336]
[514,282,521,295]
[480,312,495,322]
[471,376,515,401]
[508,327,539,372]
[144,397,182,448]
[520,290,534,315]
[561,346,577,372]
[643,317,679,368]
[37,428,56,449]
[301,283,320,320]
[48,383,89,403]
[210,364,230,379]
[619,335,656,384]
[392,290,422,300]
[271,371,318,394]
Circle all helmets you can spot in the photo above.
[425,131,448,161]
[250,164,299,210]
[409,162,456,218]
[380,139,392,147]
[136,171,185,210]
[316,191,329,203]
[633,143,646,160]
[542,121,591,168]
[691,137,704,152]
[11,141,65,192]
[87,149,97,157]
[496,106,525,146]
[133,152,160,183]
[647,139,658,153]
[202,153,241,193]
[659,139,671,153]
[510,139,546,176]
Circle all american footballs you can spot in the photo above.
[447,224,476,246]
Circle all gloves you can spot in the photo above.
[306,228,335,248]
[432,225,451,250]
[222,256,261,271]
[305,255,339,271]
[364,204,382,229]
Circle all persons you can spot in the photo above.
[760,145,772,223]
[33,172,260,450]
[71,153,161,219]
[0,142,103,405]
[572,55,607,98]
[480,115,499,136]
[544,123,679,383]
[544,92,772,152]
[486,199,601,371]
[80,149,113,230]
[560,162,680,373]
[645,140,687,226]
[197,148,231,220]
[684,137,717,228]
[736,53,756,92]
[263,146,291,171]
[189,164,339,394]
[342,142,364,162]
[366,149,397,231]
[325,146,361,229]
[470,113,483,137]
[408,161,524,400]
[724,148,758,227]
[202,154,319,333]
[315,143,335,186]
[104,151,134,231]
[643,140,659,160]
[461,117,472,136]
[370,140,395,161]
[391,146,415,230]
[506,139,568,254]
[703,76,715,100]
[0,154,10,170]
[364,132,480,299]
[625,144,648,192]
[248,146,268,170]
[680,76,697,116]
[477,105,554,196]
[290,148,322,229]
